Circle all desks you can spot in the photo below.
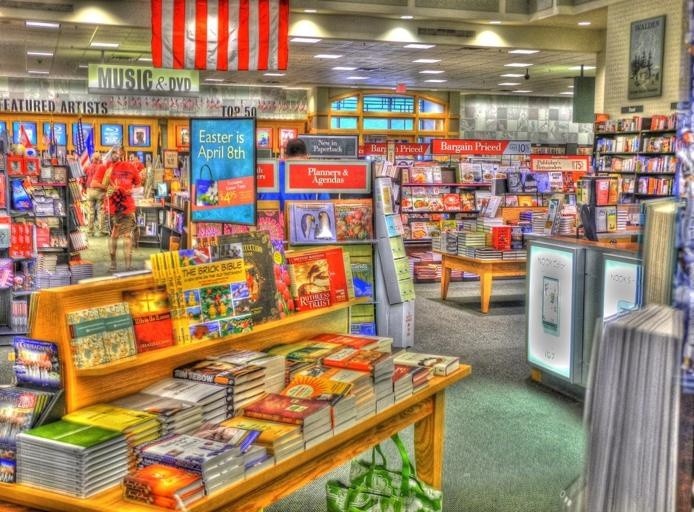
[432,247,525,315]
[0,339,473,512]
[133,197,163,251]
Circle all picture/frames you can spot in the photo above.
[627,15,666,100]
[71,123,151,148]
[176,125,191,148]
[256,127,299,151]
[0,122,66,147]
[188,117,258,227]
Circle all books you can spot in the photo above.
[0,113,694,512]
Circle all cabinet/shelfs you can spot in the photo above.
[0,164,71,340]
[156,202,189,253]
[290,238,381,310]
[398,166,575,249]
[592,129,676,202]
